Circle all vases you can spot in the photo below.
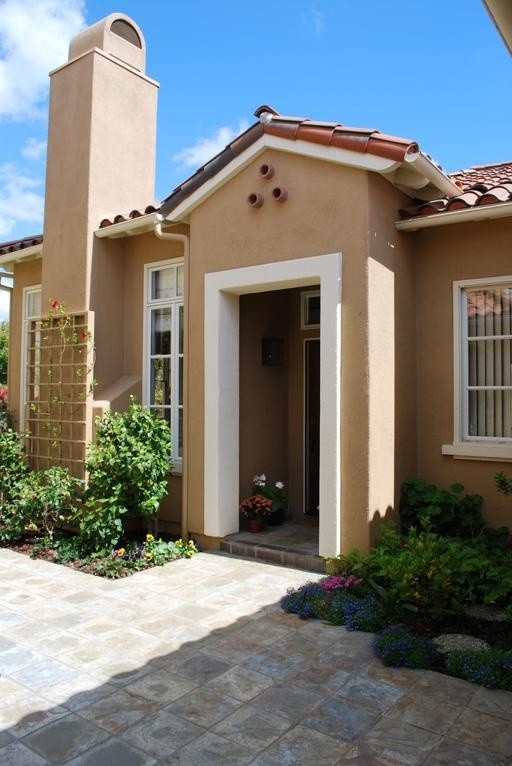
[249,520,261,532]
[267,511,283,526]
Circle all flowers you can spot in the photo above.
[240,494,272,522]
[253,472,289,511]
